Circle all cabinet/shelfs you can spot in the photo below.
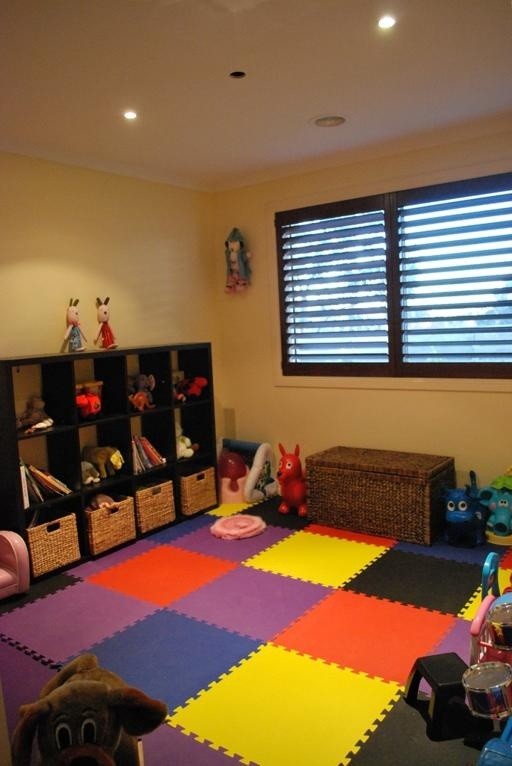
[0,341,222,586]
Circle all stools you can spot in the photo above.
[402,651,474,739]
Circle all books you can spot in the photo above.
[131,435,166,475]
[20,461,72,509]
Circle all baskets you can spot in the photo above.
[25,513,81,578]
[136,480,176,532]
[83,496,136,555]
[180,467,218,516]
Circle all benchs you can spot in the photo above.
[302,442,458,547]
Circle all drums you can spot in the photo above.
[462,662,512,722]
[487,605,512,651]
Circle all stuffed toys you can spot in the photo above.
[93,296,118,348]
[83,445,125,479]
[9,653,167,766]
[128,373,156,411]
[223,226,253,292]
[20,395,54,433]
[172,376,206,401]
[175,421,198,460]
[73,380,104,420]
[64,296,88,351]
